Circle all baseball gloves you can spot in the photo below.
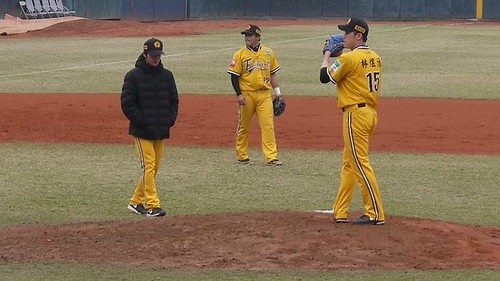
[273,95,286,117]
[321,33,345,57]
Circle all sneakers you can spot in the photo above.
[237,158,253,165]
[146,207,166,217]
[127,203,147,215]
[267,159,282,166]
[334,218,349,223]
[352,214,386,225]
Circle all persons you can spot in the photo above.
[228,24,284,166]
[121,38,179,217]
[320,16,385,225]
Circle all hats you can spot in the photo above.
[338,16,369,37]
[240,23,263,37]
[143,38,165,57]
